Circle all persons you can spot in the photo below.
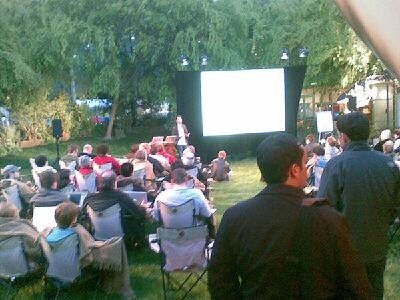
[0,202,44,276]
[82,169,149,250]
[208,131,378,300]
[172,116,190,158]
[211,150,231,181]
[316,112,400,300]
[153,167,216,241]
[41,201,128,294]
[298,128,399,199]
[0,140,209,218]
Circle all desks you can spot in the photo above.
[149,141,176,153]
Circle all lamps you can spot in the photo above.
[180,47,309,66]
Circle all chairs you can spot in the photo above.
[0,156,211,300]
[152,135,177,142]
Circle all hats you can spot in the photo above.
[2,165,21,177]
